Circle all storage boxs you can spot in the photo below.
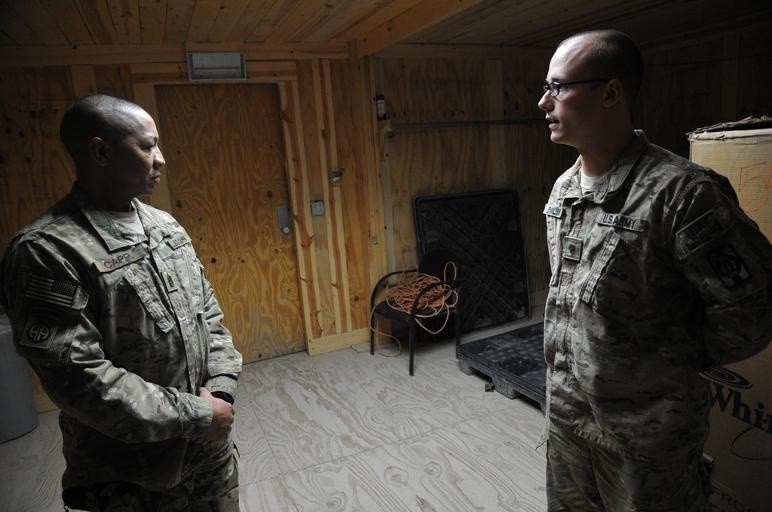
[689,115,772,511]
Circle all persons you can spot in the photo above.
[537,30,771,512]
[1,94,243,512]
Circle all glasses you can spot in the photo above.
[540,76,626,97]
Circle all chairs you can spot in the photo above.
[369,250,466,376]
